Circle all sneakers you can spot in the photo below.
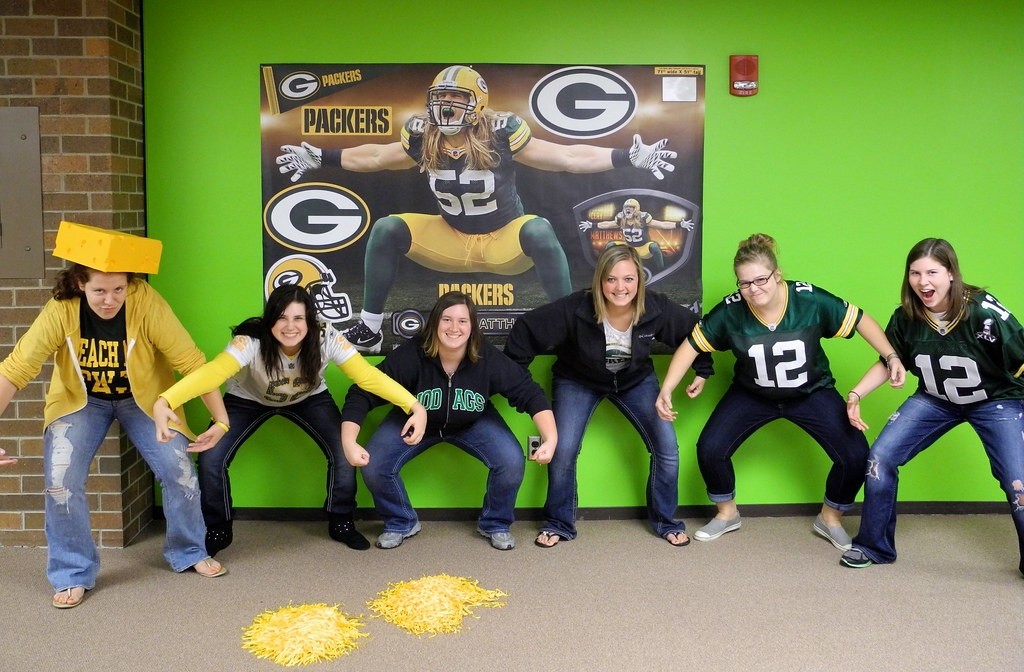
[841,548,872,568]
[695,510,742,542]
[477,516,515,550]
[341,318,384,355]
[812,513,852,551]
[375,520,422,549]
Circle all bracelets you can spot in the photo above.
[886,353,900,362]
[214,420,229,434]
[849,390,860,403]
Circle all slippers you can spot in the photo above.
[53,586,83,607]
[666,531,691,546]
[534,531,560,547]
[195,556,227,577]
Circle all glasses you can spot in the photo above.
[736,267,776,290]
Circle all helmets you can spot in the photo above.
[623,199,640,219]
[425,65,488,134]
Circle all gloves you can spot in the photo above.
[578,220,592,232]
[623,134,678,181]
[276,141,322,182]
[680,218,695,232]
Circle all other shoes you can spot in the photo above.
[203,508,236,558]
[328,512,370,550]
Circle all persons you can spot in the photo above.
[504,244,715,548]
[276,65,679,353]
[840,238,1024,574]
[340,291,558,550]
[153,284,427,557]
[579,199,694,272]
[654,233,906,551]
[0,262,230,608]
